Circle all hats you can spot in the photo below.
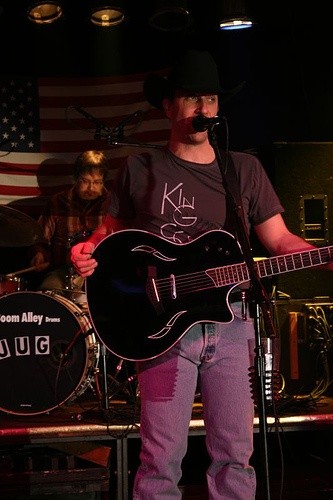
[142,51,225,109]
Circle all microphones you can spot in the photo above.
[114,110,142,132]
[73,105,111,132]
[192,115,226,132]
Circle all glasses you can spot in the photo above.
[80,177,100,185]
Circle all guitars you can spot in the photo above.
[85,229,333,362]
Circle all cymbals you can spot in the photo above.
[0,204,45,245]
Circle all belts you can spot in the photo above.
[228,290,249,303]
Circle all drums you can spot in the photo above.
[0,290,97,416]
[0,274,24,297]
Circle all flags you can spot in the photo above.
[0,72,171,205]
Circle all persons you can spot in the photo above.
[68,46,333,500]
[31,150,121,300]
